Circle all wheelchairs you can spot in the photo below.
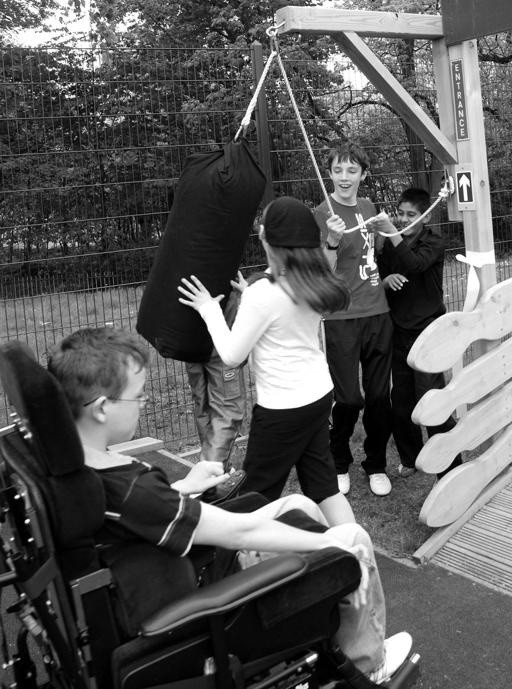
[2,338,420,689]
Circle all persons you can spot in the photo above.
[309,141,390,495]
[378,187,464,485]
[184,281,247,477]
[44,325,413,685]
[177,195,358,526]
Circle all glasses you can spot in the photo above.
[83,392,150,411]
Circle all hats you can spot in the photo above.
[260,196,320,249]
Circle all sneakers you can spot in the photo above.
[337,471,350,494]
[369,473,392,496]
[366,631,413,685]
[398,464,417,478]
